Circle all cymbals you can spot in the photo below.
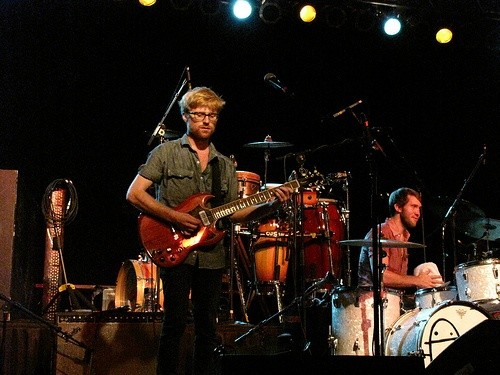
[336,239,427,248]
[464,218,500,241]
[245,141,293,148]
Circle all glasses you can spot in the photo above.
[182,112,219,122]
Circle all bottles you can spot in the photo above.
[144,276,155,312]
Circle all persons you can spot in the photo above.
[358,188,445,293]
[124,87,292,375]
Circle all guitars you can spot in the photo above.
[136,168,327,267]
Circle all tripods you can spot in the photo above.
[39,209,96,318]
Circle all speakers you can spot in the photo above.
[53,312,500,375]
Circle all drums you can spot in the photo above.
[235,171,261,235]
[329,287,403,357]
[293,188,318,209]
[384,300,492,368]
[301,198,346,294]
[414,285,458,308]
[114,259,194,315]
[252,183,299,287]
[454,257,500,315]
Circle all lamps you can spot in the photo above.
[137,0,282,24]
[292,2,405,43]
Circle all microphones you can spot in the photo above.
[187,66,191,90]
[483,144,486,164]
[264,73,288,92]
[84,346,92,365]
[327,272,341,288]
[332,100,363,117]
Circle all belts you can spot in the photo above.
[359,285,402,297]
